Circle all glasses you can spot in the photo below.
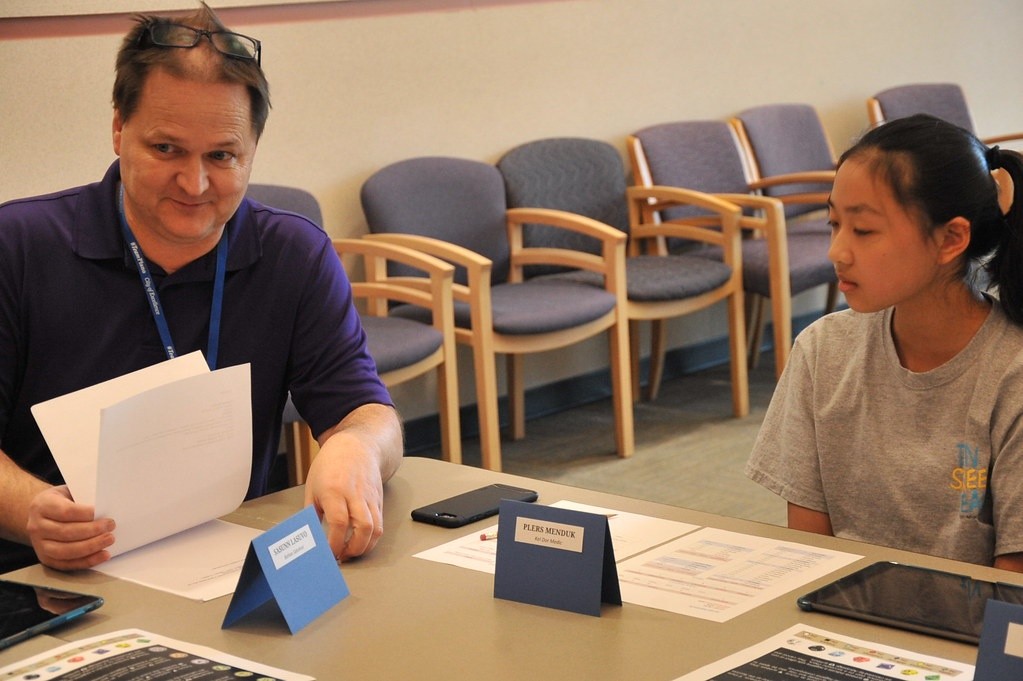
[135,20,261,68]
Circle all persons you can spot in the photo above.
[743,113,1023,572]
[0,2,404,570]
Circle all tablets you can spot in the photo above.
[0,580,105,652]
[797,560,1023,647]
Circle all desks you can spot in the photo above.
[0,456,1023,681]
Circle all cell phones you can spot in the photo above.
[411,483,539,529]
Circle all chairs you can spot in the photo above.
[243,81,1023,488]
